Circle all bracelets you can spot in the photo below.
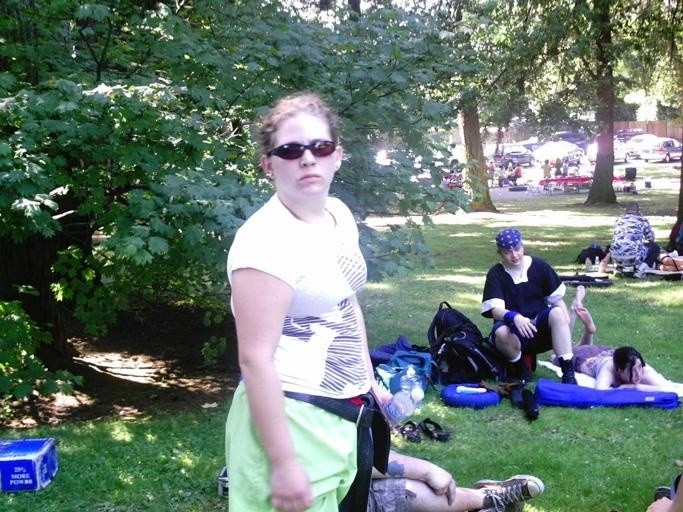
[503,311,517,321]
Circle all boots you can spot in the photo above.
[510,353,532,385]
[558,356,577,385]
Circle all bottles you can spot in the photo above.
[585,257,592,272]
[383,387,424,431]
[594,256,600,271]
[398,364,423,407]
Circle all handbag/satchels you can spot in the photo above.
[578,248,605,263]
[372,334,444,392]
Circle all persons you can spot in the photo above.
[602,243,683,272]
[226,93,393,511]
[608,201,660,279]
[552,284,675,392]
[480,229,578,409]
[543,157,595,189]
[646,471,683,512]
[219,450,545,512]
[486,161,522,187]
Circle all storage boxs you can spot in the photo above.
[0,437,59,495]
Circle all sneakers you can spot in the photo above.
[470,475,544,511]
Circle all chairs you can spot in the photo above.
[438,160,637,199]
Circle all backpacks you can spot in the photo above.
[428,302,503,384]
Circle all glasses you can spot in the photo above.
[267,141,336,160]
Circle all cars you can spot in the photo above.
[615,128,643,142]
[626,133,658,157]
[534,142,582,166]
[518,130,587,152]
[586,140,629,164]
[491,142,534,167]
[637,136,682,163]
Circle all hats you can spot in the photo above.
[496,229,522,251]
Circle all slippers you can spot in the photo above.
[399,421,421,443]
[419,418,450,442]
